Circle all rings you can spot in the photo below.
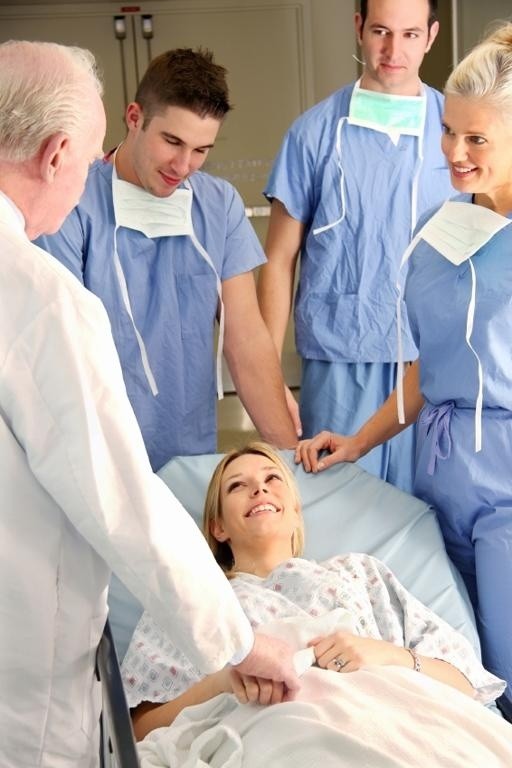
[332,657,345,667]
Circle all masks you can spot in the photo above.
[348,88,427,137]
[419,202,510,267]
[111,178,194,239]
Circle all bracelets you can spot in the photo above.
[405,648,422,673]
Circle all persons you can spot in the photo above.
[0,34,304,768]
[294,14,512,724]
[32,43,299,484]
[259,1,472,497]
[116,438,504,742]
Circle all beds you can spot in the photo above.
[97,446,511,768]
